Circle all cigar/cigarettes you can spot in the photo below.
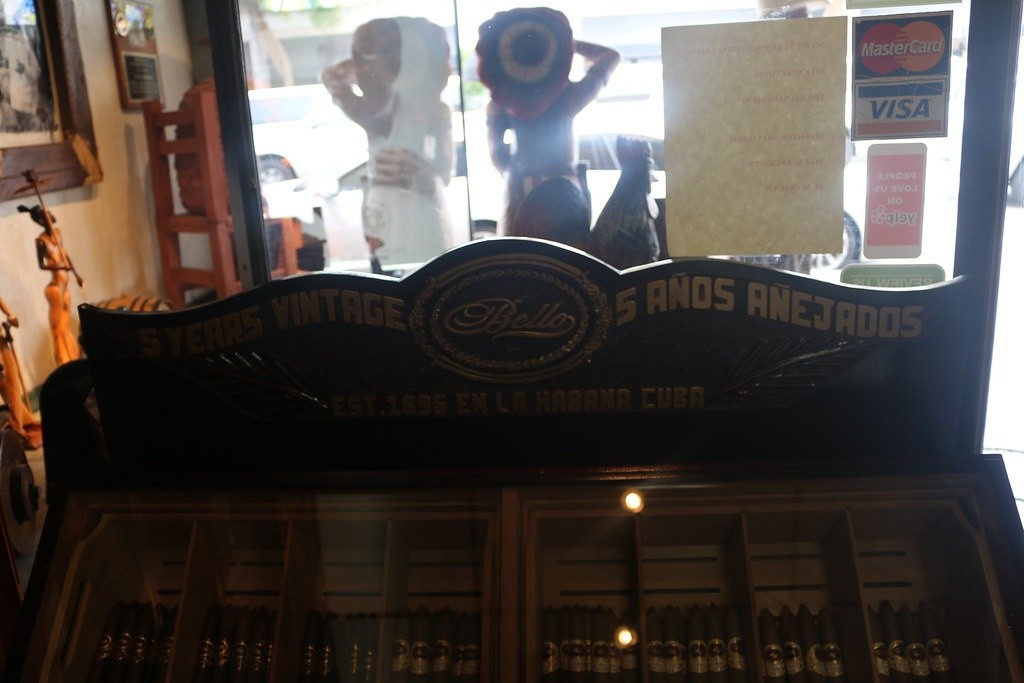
[88,597,956,682]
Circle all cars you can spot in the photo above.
[245,76,871,277]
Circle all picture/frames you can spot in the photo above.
[0,1,104,200]
[107,0,167,111]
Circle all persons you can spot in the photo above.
[477,7,620,235]
[0,14,40,134]
[0,300,41,437]
[322,18,452,277]
[16,205,84,368]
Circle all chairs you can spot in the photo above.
[142,78,299,307]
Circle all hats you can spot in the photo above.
[476,8,574,120]
[352,17,451,123]
